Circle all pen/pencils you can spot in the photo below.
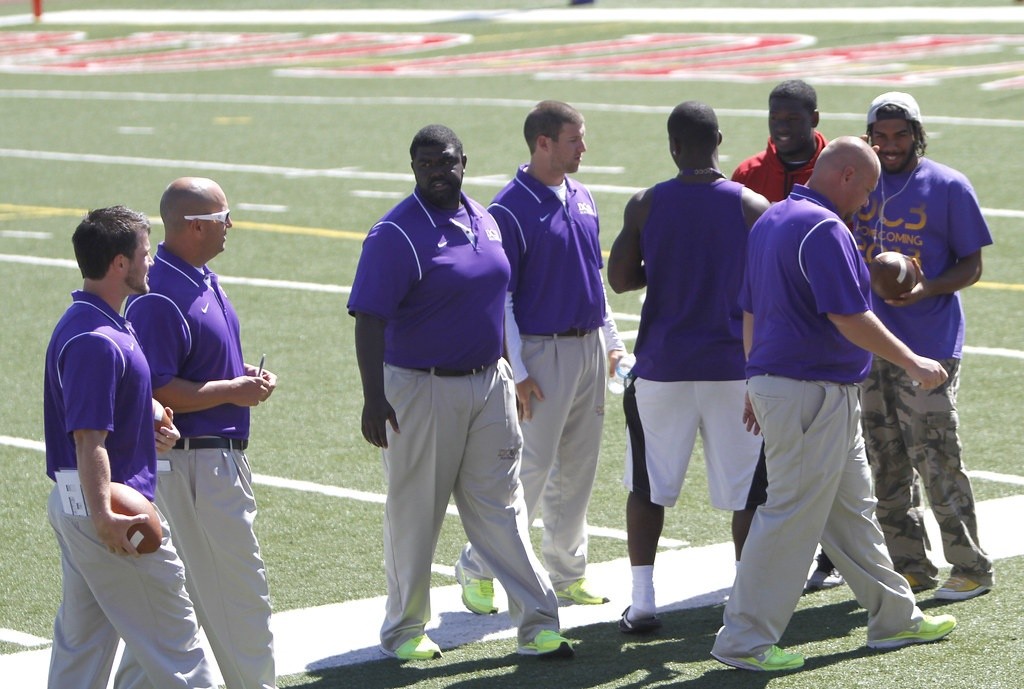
[912,381,921,388]
[257,353,266,376]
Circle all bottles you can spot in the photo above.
[608,354,637,394]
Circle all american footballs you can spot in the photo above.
[108,481,164,552]
[151,397,173,442]
[869,251,917,300]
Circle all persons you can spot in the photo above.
[346,123,576,660]
[606,102,771,633]
[710,135,958,671]
[454,99,628,614]
[44,172,278,689]
[852,92,995,600]
[731,80,846,587]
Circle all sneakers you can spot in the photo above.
[805,565,845,591]
[934,576,988,600]
[709,644,805,671]
[454,560,498,615]
[516,629,574,656]
[555,577,610,605]
[380,633,444,661]
[619,605,662,632]
[897,571,928,590]
[866,615,957,648]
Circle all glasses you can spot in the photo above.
[184,208,230,223]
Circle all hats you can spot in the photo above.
[866,91,922,127]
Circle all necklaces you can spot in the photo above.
[677,167,726,178]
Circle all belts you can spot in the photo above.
[537,328,589,338]
[417,364,485,376]
[172,437,248,450]
